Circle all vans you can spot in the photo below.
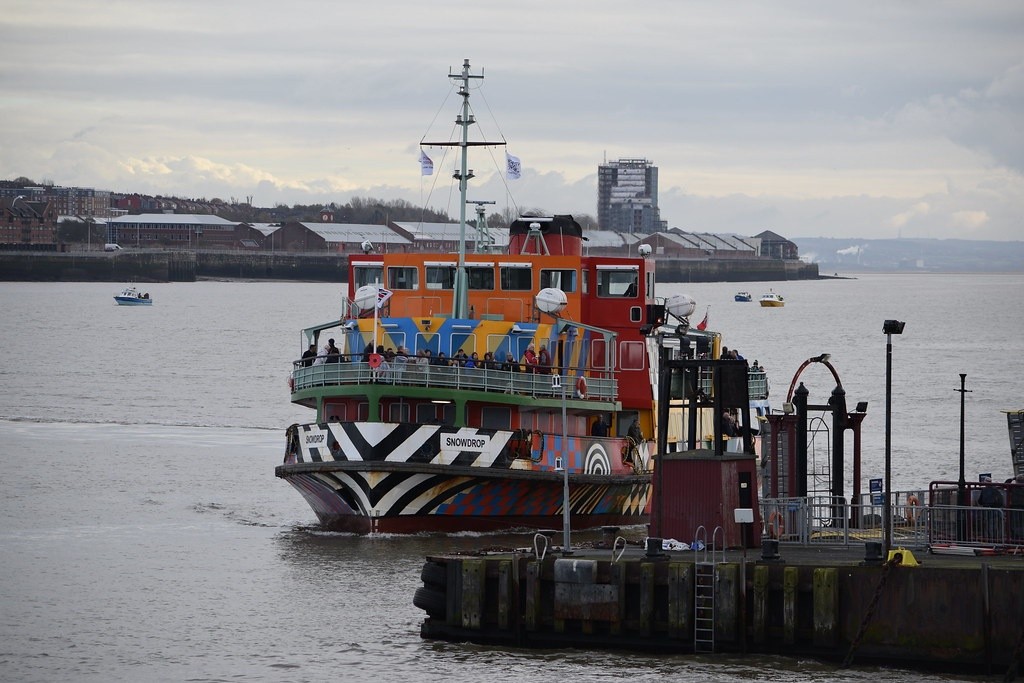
[104,244,123,252]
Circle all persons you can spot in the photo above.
[720,347,765,380]
[722,412,741,438]
[591,414,607,437]
[623,277,637,298]
[301,338,551,392]
[626,419,643,461]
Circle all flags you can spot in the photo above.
[421,150,434,175]
[507,153,522,180]
[697,314,709,330]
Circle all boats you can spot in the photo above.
[272,57,770,535]
[734,291,752,302]
[113,289,153,305]
[759,288,785,307]
[927,542,1006,556]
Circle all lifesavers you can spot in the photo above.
[906,496,921,526]
[769,512,784,539]
[576,376,587,399]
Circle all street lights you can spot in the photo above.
[884,319,905,558]
